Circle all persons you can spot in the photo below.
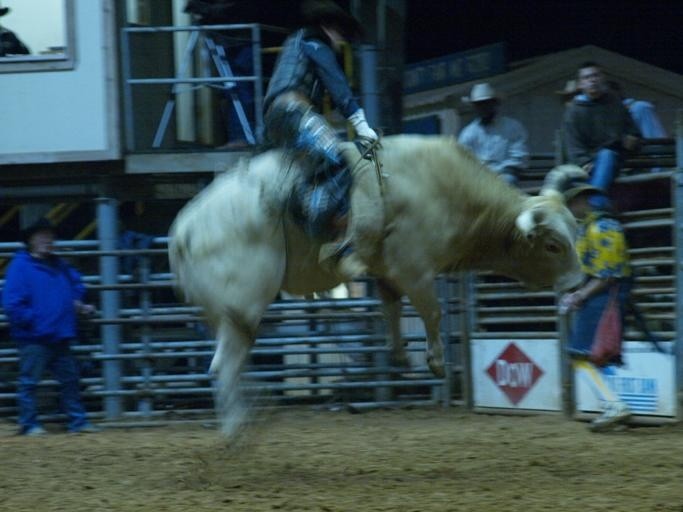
[552,76,584,166]
[552,178,636,432]
[452,80,530,190]
[0,218,105,438]
[605,78,673,174]
[257,1,384,281]
[562,58,646,215]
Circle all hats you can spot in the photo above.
[18,214,55,243]
[555,80,579,95]
[543,164,608,201]
[461,82,498,106]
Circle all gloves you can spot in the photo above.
[347,108,378,140]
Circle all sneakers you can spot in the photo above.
[589,401,630,432]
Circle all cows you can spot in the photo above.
[166,134,591,442]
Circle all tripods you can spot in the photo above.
[152,24,257,150]
